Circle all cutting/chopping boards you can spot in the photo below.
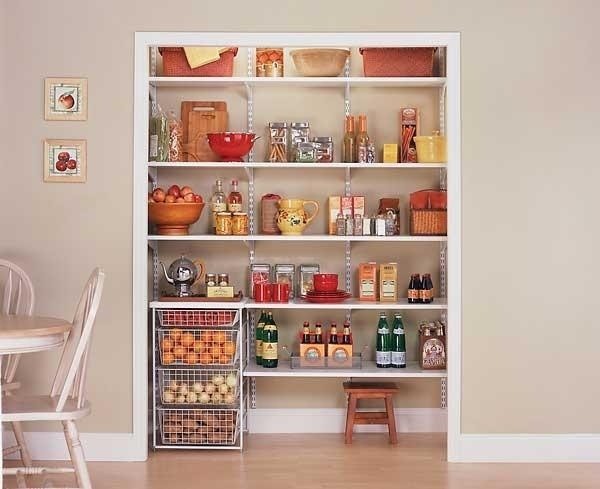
[180,100,228,162]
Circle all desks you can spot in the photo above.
[1,313,72,488]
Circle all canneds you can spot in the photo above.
[215,212,231,236]
[250,263,270,299]
[297,136,334,163]
[275,263,296,300]
[231,212,249,236]
[298,264,320,299]
[218,273,229,287]
[205,273,216,287]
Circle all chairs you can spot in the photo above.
[2,265,106,488]
[1,259,34,472]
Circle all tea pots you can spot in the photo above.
[273,198,319,235]
[157,253,205,298]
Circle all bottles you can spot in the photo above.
[255,310,278,368]
[300,319,351,344]
[376,312,406,366]
[149,105,184,163]
[210,176,249,236]
[407,273,434,303]
[341,113,369,162]
[335,213,394,235]
[206,273,228,286]
[418,320,445,337]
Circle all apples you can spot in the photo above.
[58,94,74,109]
[148,185,202,203]
[166,311,231,325]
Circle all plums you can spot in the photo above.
[56,152,76,171]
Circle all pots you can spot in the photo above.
[411,130,447,164]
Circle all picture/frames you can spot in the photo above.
[44,76,90,121]
[42,138,89,184]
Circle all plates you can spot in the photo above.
[304,290,352,303]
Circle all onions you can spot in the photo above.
[163,374,237,404]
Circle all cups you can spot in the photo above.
[254,282,289,302]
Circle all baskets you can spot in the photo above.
[158,366,240,406]
[156,309,239,327]
[156,327,239,366]
[157,405,240,446]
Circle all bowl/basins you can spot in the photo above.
[312,273,339,291]
[289,48,351,77]
[205,132,261,161]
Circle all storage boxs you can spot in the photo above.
[360,46,439,76]
[159,46,238,77]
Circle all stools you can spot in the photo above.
[342,381,400,447]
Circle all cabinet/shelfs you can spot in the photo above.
[134,30,462,381]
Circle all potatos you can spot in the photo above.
[164,411,236,443]
